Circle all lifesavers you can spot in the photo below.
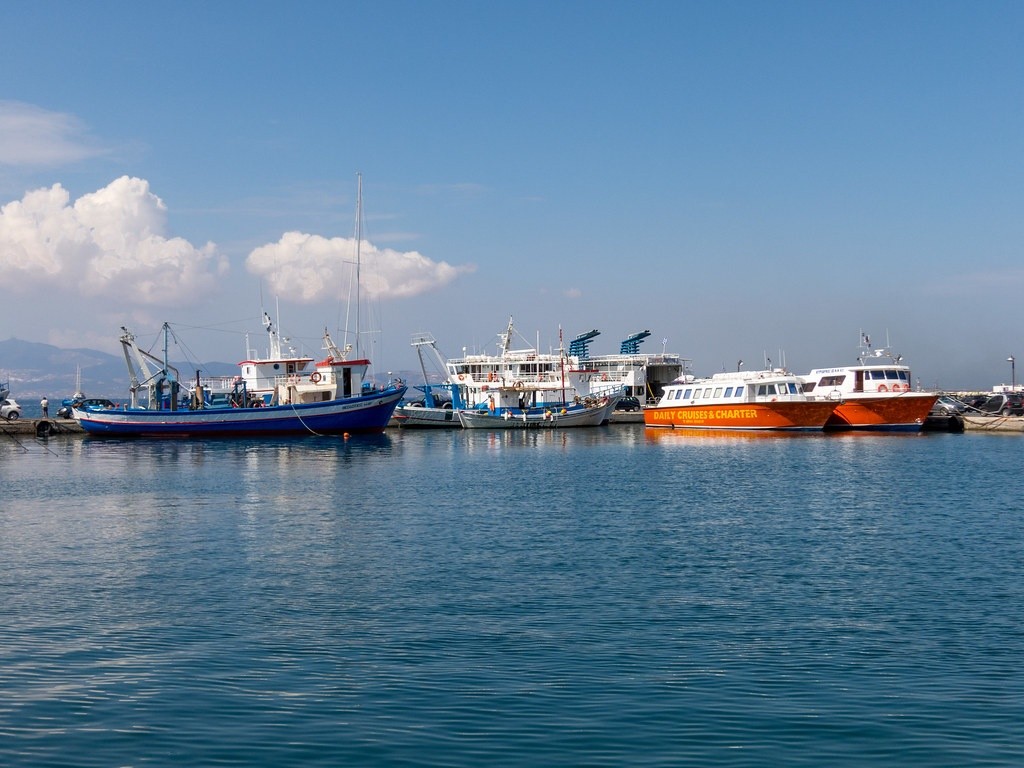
[877,384,889,392]
[35,419,53,438]
[902,383,910,392]
[311,372,321,382]
[891,383,900,392]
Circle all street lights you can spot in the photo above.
[1006,355,1015,393]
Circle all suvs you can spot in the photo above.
[615,396,641,412]
[979,394,1024,417]
[0,399,22,420]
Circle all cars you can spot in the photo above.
[930,397,966,416]
[55,398,115,419]
[960,395,989,414]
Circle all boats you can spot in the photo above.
[71,283,411,441]
[644,344,847,434]
[804,328,943,434]
[393,314,626,428]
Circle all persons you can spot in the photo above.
[40,397,49,419]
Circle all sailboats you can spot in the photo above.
[60,364,89,406]
